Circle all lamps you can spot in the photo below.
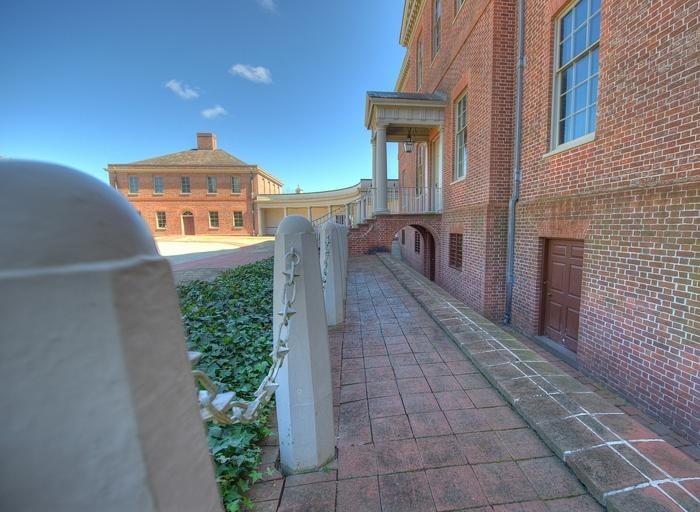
[403,128,415,152]
